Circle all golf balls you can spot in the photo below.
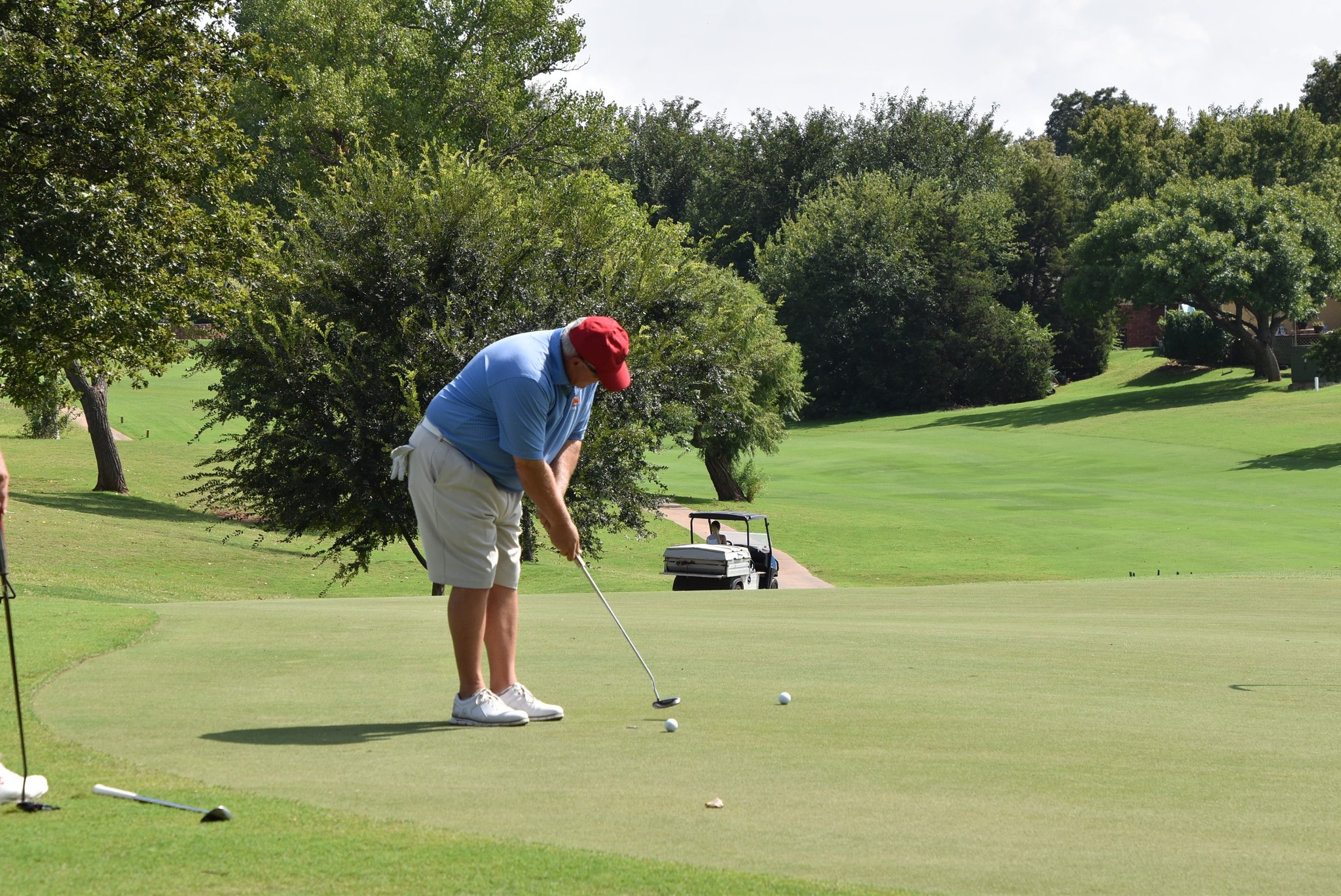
[665,719,679,732]
[778,692,791,704]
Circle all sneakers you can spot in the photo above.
[497,682,564,721]
[450,688,530,728]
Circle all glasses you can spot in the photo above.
[581,358,602,384]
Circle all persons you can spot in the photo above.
[408,317,630,726]
[0,446,48,800]
[706,521,727,545]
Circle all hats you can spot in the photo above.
[569,316,630,392]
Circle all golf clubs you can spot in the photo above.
[0,515,62,813]
[93,783,234,824]
[572,553,682,710]
